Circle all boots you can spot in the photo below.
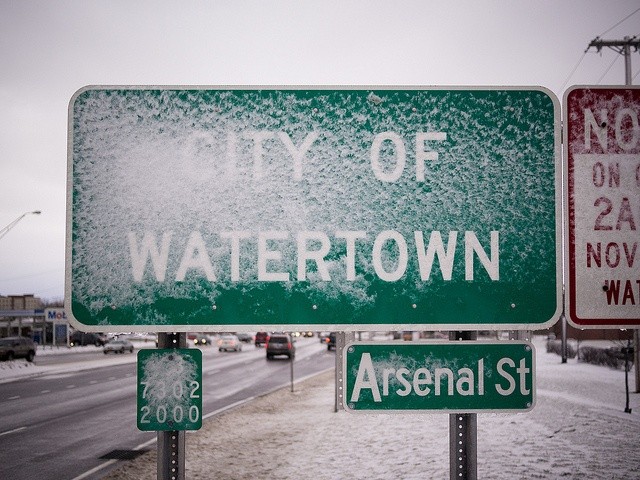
[0,338,35,362]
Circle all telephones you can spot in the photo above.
[563,86,640,330]
[342,344,534,410]
[136,347,201,431]
[66,87,563,332]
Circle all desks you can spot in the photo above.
[0,209,44,241]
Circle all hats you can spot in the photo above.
[317,333,338,351]
[104,341,134,354]
[195,337,211,346]
[267,337,294,361]
[255,333,268,347]
[66,333,103,346]
[219,336,242,352]
